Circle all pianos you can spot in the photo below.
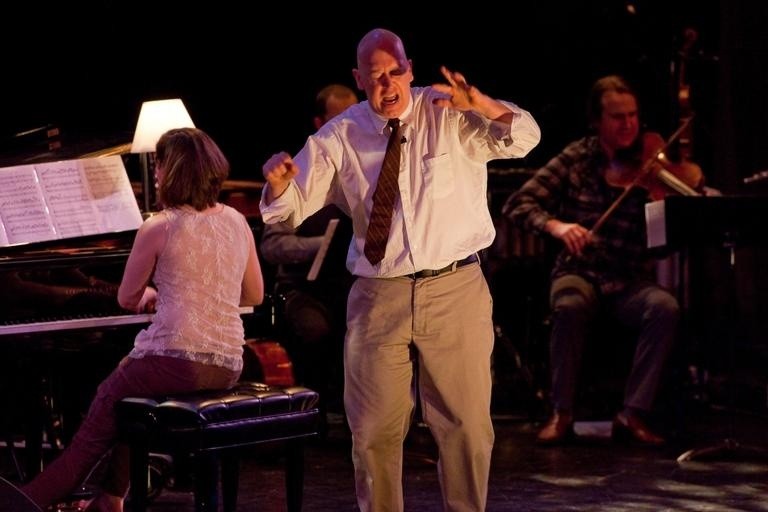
[3,236,292,511]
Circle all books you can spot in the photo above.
[0,154,145,249]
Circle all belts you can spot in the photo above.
[407,252,477,279]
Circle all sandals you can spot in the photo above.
[83,495,124,512]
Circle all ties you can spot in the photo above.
[364,118,403,267]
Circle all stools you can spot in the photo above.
[115,382,320,512]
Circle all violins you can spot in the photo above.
[605,133,704,202]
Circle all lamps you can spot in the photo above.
[130,97,198,156]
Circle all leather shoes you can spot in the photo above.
[613,412,665,445]
[536,409,574,443]
[327,413,346,439]
[407,425,437,466]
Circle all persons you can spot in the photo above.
[17,126,266,510]
[256,86,360,439]
[256,26,542,511]
[498,72,723,450]
